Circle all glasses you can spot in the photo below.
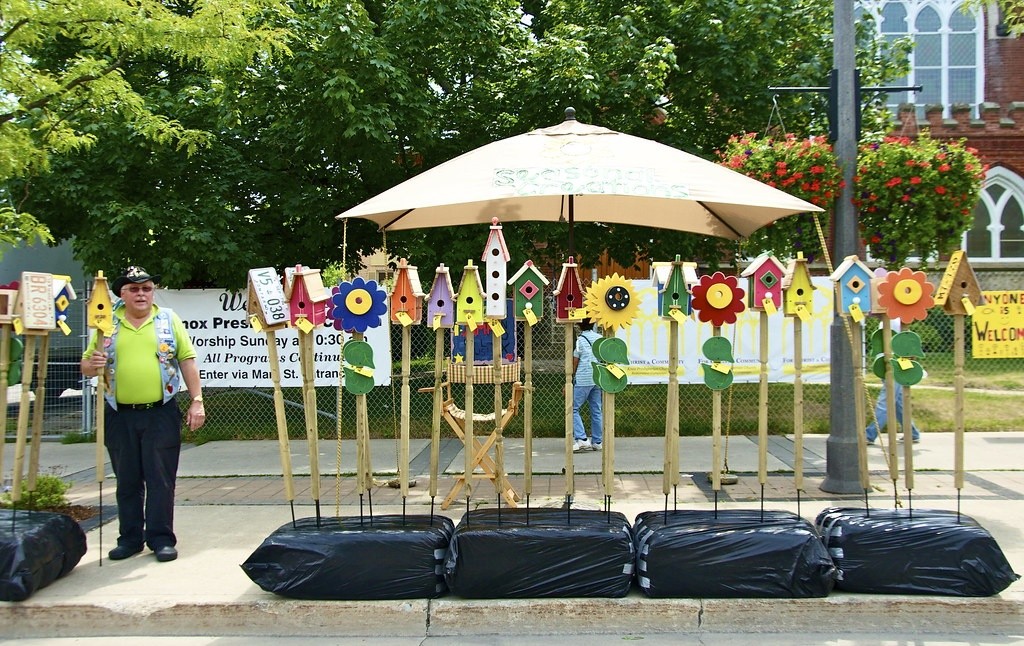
[122,285,154,293]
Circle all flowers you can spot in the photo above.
[713,130,845,266]
[854,136,989,277]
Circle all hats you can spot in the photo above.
[111,265,162,298]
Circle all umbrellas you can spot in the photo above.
[330,107,827,258]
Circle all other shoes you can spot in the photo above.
[866,437,875,444]
[897,434,920,442]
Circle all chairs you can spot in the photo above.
[416,357,524,509]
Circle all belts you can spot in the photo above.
[117,399,164,409]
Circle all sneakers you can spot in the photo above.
[573,438,592,452]
[592,442,603,452]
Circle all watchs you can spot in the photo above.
[192,395,203,402]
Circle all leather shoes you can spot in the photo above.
[109,543,144,560]
[154,544,177,561]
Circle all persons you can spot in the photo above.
[78,267,206,561]
[572,318,603,451]
[865,315,920,445]
